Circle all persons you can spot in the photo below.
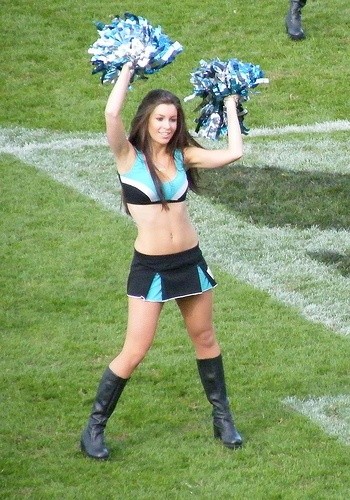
[285,0,307,40]
[80,62,243,461]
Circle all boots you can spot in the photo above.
[196,354,243,446]
[80,365,131,460]
[286,0,307,40]
